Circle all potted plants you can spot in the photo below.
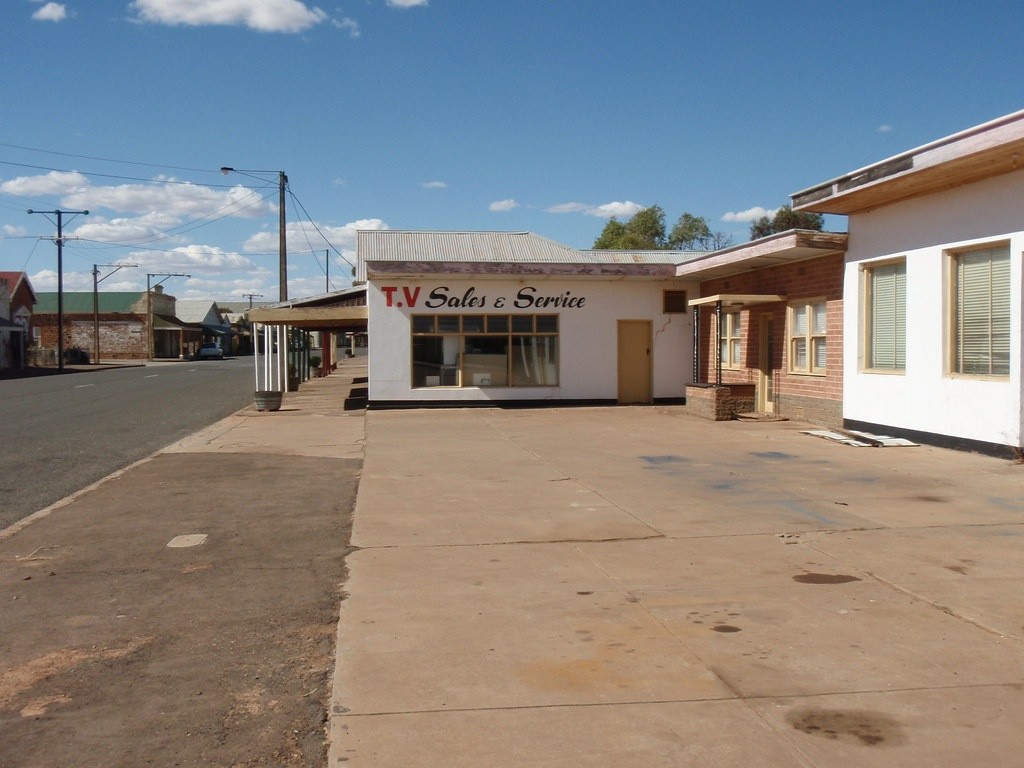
[345,348,352,359]
[278,363,300,392]
[308,356,324,377]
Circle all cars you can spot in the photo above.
[198,342,224,359]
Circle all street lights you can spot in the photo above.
[220,166,288,304]
